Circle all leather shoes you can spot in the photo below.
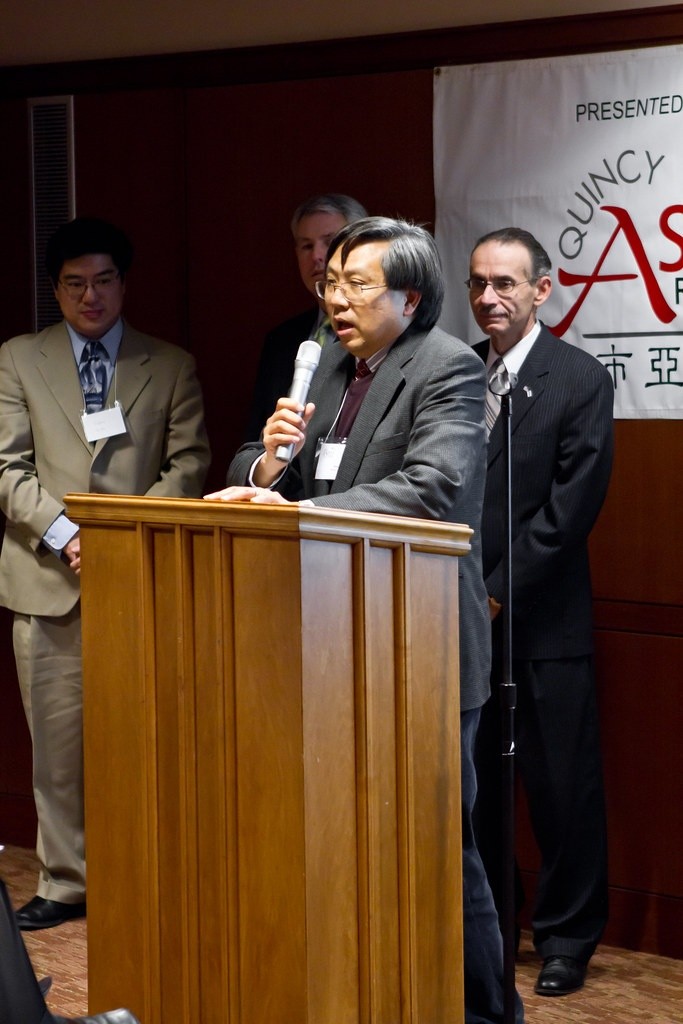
[534,955,588,994]
[12,895,87,932]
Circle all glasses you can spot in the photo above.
[463,276,536,293]
[57,273,119,296]
[315,281,395,302]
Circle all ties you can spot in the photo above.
[485,359,507,437]
[354,360,372,381]
[78,341,108,446]
[312,315,331,347]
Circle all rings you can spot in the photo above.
[255,491,259,496]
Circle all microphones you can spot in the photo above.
[275,341,322,464]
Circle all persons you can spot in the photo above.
[0,216,214,929]
[204,189,616,1024]
